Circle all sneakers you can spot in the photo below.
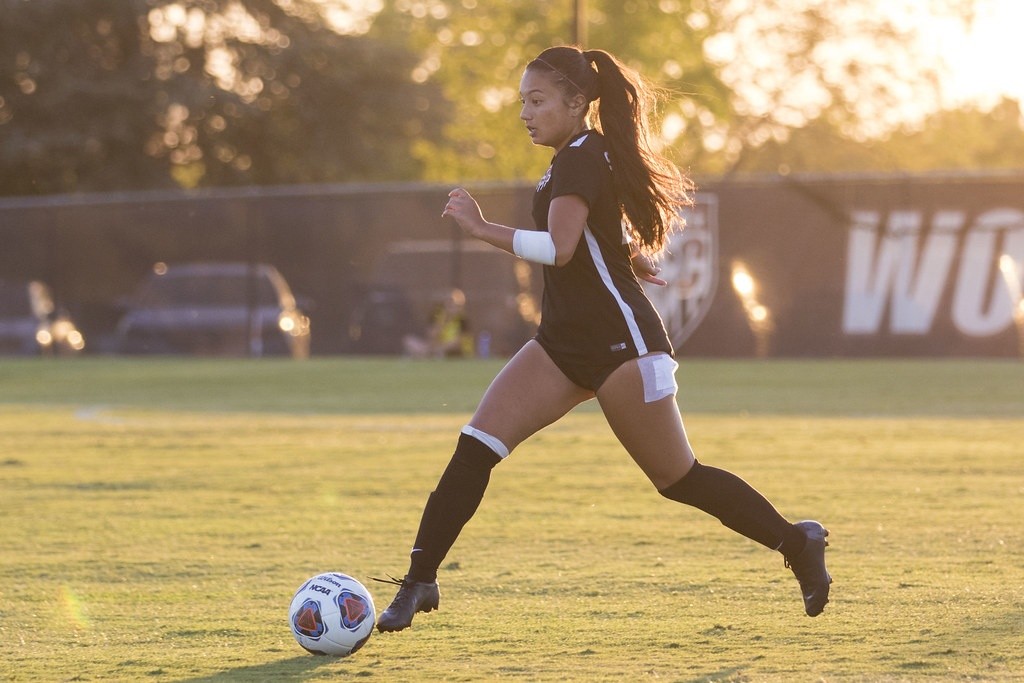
[782,520,834,617]
[365,571,440,634]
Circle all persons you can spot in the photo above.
[366,47,835,633]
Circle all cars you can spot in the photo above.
[0,277,71,357]
[116,260,311,358]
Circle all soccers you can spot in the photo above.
[290,574,375,659]
